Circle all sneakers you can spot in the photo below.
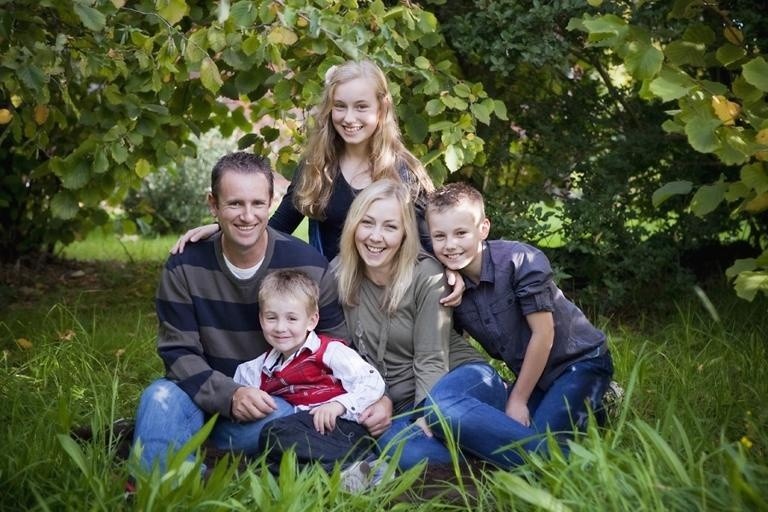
[332,459,374,499]
[604,381,624,421]
[367,459,397,488]
[67,415,134,443]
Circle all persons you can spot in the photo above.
[231,269,397,495]
[72,152,357,486]
[170,60,466,308]
[425,182,614,441]
[327,178,570,477]
